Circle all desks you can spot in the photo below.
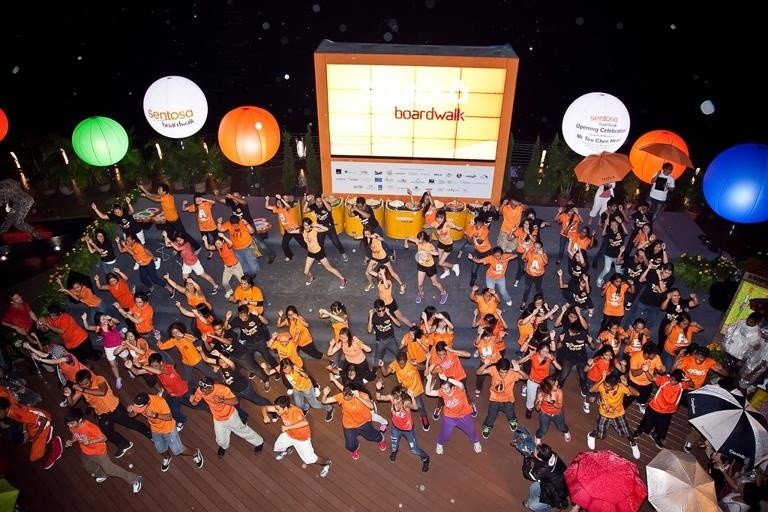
[421,199,466,241]
[301,195,344,235]
[464,201,497,245]
[384,200,426,240]
[276,195,302,236]
[344,195,384,240]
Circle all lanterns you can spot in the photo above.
[142,75,209,141]
[0,109,9,140]
[217,104,282,169]
[629,129,689,185]
[561,90,632,156]
[702,141,768,225]
[71,116,131,168]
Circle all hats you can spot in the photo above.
[47,304,62,314]
[132,392,149,414]
[343,384,357,401]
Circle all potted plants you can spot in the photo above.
[624,177,638,209]
[48,161,78,196]
[206,143,232,194]
[158,144,189,190]
[557,168,573,207]
[191,154,208,192]
[123,147,153,191]
[91,166,111,192]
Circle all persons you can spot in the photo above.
[321,163,767,473]
[1,178,348,496]
[718,471,760,511]
[519,443,570,511]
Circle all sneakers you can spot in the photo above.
[509,418,517,432]
[130,284,136,294]
[325,408,333,423]
[153,257,161,271]
[192,448,204,469]
[128,370,135,380]
[59,398,68,408]
[473,441,482,454]
[506,299,513,308]
[520,384,528,397]
[329,354,337,370]
[351,450,360,460]
[302,409,310,417]
[255,444,263,454]
[630,441,640,460]
[435,442,444,455]
[305,276,314,286]
[133,476,142,494]
[224,288,234,299]
[457,249,463,261]
[470,402,478,418]
[284,256,295,261]
[114,442,133,458]
[275,448,294,460]
[651,434,664,449]
[313,382,321,398]
[581,387,587,397]
[194,245,203,257]
[176,417,188,432]
[171,249,179,256]
[271,413,278,422]
[380,422,389,434]
[275,373,282,381]
[389,250,396,263]
[624,301,632,311]
[115,377,122,391]
[52,437,63,455]
[684,439,692,454]
[415,290,424,304]
[41,363,56,374]
[362,255,371,266]
[339,278,348,289]
[341,253,349,262]
[399,283,407,295]
[389,451,396,463]
[372,365,379,373]
[519,301,526,312]
[639,402,646,415]
[592,257,599,267]
[596,278,604,288]
[475,389,481,398]
[525,407,533,419]
[364,282,375,292]
[96,477,108,483]
[42,453,62,470]
[161,454,172,472]
[555,258,561,266]
[439,291,449,305]
[422,457,430,473]
[207,251,214,260]
[149,436,154,444]
[286,388,294,395]
[169,287,175,299]
[420,416,430,432]
[534,435,541,448]
[144,287,154,296]
[588,308,593,318]
[320,460,332,478]
[268,254,277,264]
[133,262,140,271]
[481,425,492,440]
[586,431,596,451]
[452,264,460,277]
[217,446,225,459]
[583,400,591,414]
[378,441,387,452]
[470,278,477,286]
[563,430,571,444]
[513,280,519,287]
[264,380,271,392]
[522,499,530,509]
[249,372,256,379]
[211,285,220,295]
[440,269,451,279]
[241,414,250,424]
[432,407,441,421]
[633,429,642,439]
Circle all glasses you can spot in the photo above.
[376,308,385,312]
[356,205,363,208]
[669,376,680,384]
[198,380,215,390]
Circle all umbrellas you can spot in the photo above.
[572,151,634,187]
[644,447,717,512]
[745,296,768,317]
[638,144,697,170]
[562,450,647,512]
